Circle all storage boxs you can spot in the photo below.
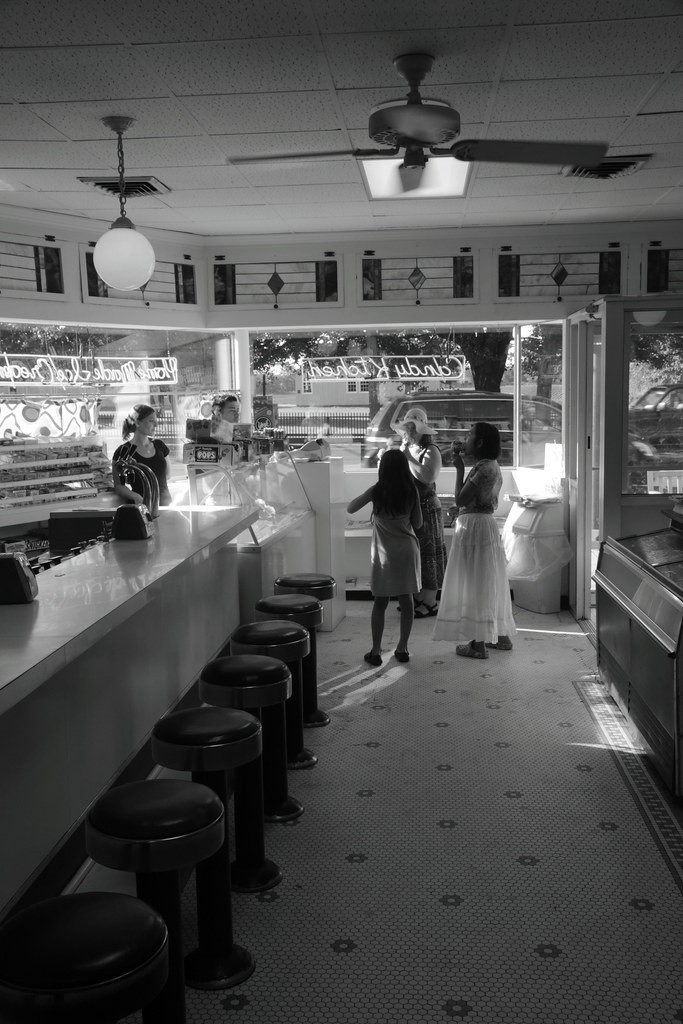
[183,395,289,466]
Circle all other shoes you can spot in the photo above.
[395,650,409,662]
[364,652,382,666]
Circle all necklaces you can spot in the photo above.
[139,443,150,453]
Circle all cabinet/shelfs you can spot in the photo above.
[0,442,114,528]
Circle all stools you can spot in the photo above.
[274,572,337,727]
[198,654,293,893]
[0,892,170,1024]
[253,594,324,770]
[229,620,311,821]
[85,778,226,1024]
[152,706,263,989]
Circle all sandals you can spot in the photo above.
[397,597,421,612]
[413,601,438,619]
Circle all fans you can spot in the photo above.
[225,52,611,193]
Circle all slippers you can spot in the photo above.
[485,638,514,651]
[456,640,488,658]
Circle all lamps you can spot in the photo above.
[92,115,155,291]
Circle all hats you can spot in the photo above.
[393,408,439,439]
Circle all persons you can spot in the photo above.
[432,423,518,659]
[392,408,448,619]
[112,404,174,507]
[347,449,423,665]
[210,395,240,424]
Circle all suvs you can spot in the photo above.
[360,384,682,466]
